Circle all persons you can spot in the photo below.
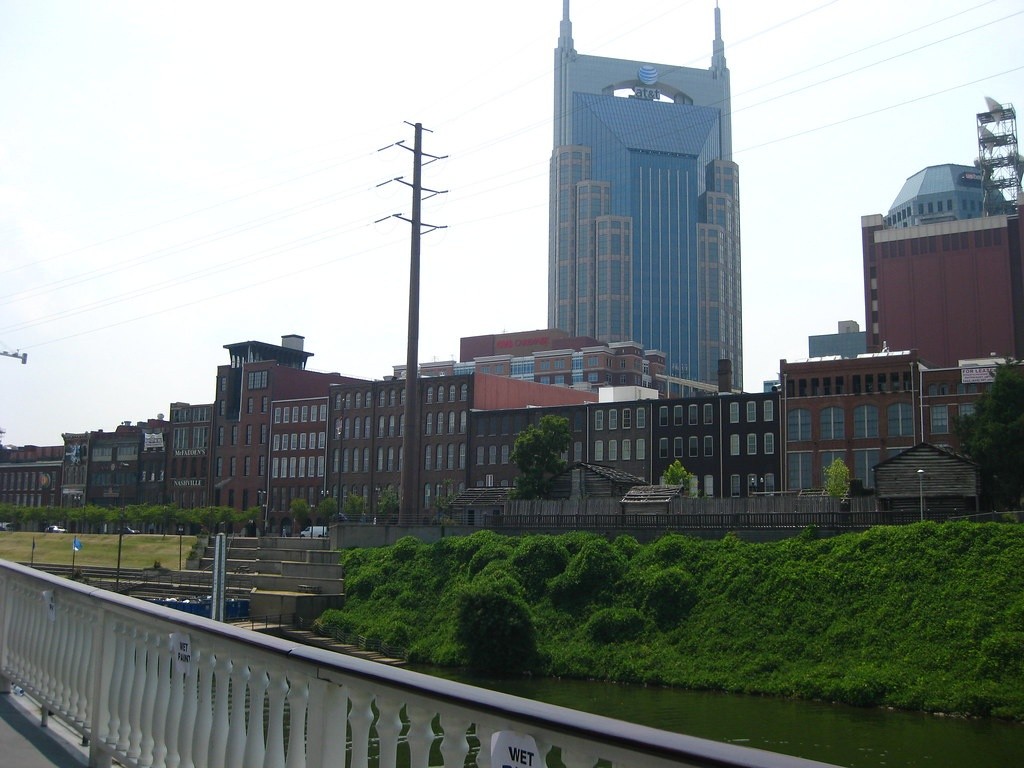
[282,527,286,537]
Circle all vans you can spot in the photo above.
[301,525,328,537]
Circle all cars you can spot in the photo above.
[44,525,69,534]
[115,526,141,534]
[0,522,14,531]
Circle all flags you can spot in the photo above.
[72,539,82,552]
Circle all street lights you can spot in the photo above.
[178,525,184,570]
[917,468,926,523]
[74,496,81,506]
[750,476,765,495]
[321,489,329,536]
[257,490,267,537]
